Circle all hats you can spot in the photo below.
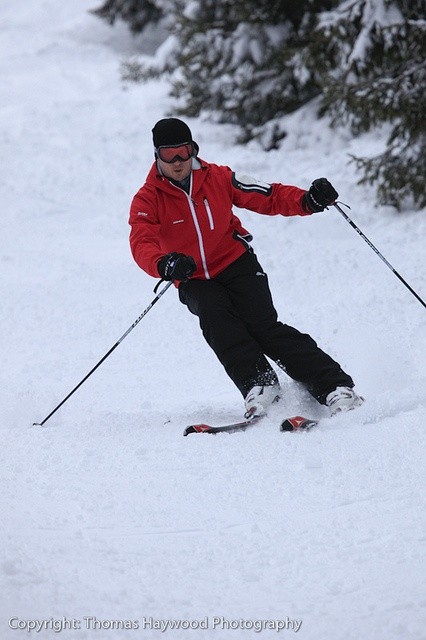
[152,118,193,149]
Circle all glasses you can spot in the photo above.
[153,141,194,164]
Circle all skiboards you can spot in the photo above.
[184,414,318,436]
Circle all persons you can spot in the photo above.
[128,119,355,419]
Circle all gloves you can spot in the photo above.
[157,252,197,282]
[305,177,339,213]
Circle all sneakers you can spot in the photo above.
[244,382,282,420]
[325,385,367,419]
